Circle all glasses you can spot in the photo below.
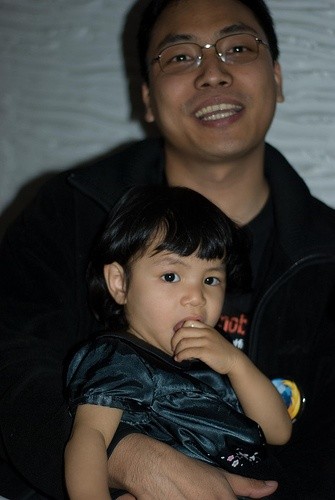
[148,33,272,76]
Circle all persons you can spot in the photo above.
[0,0,334,500]
[61,185,306,499]
